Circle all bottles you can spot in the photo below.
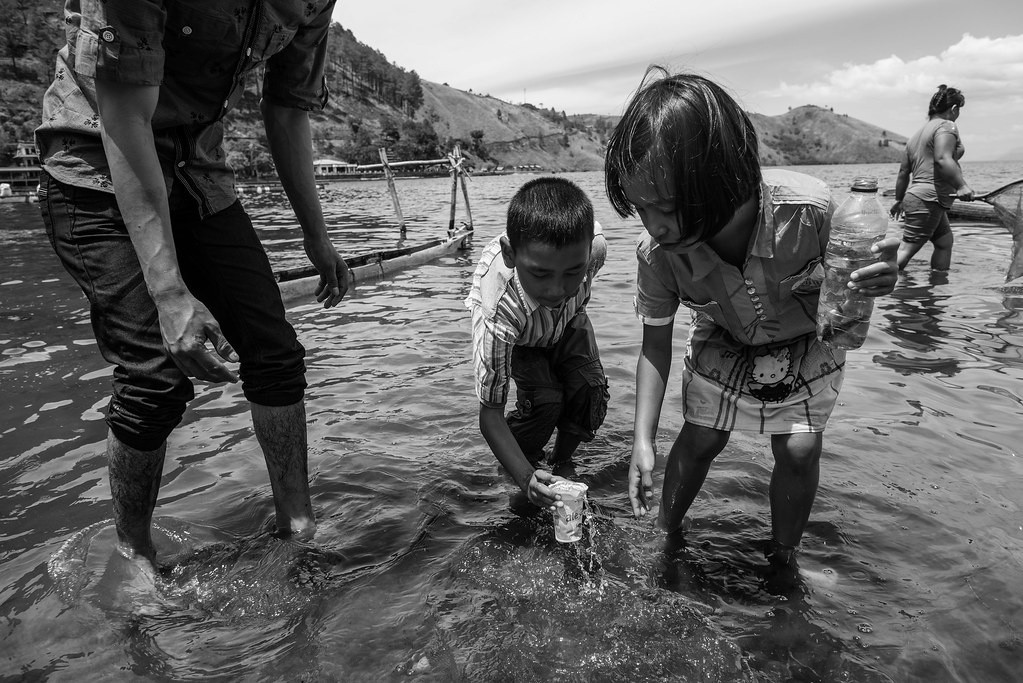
[816,177,886,350]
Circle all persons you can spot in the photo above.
[603,73,900,543]
[34,1,350,566]
[465,177,609,513]
[888,85,972,270]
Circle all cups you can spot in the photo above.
[547,481,588,544]
